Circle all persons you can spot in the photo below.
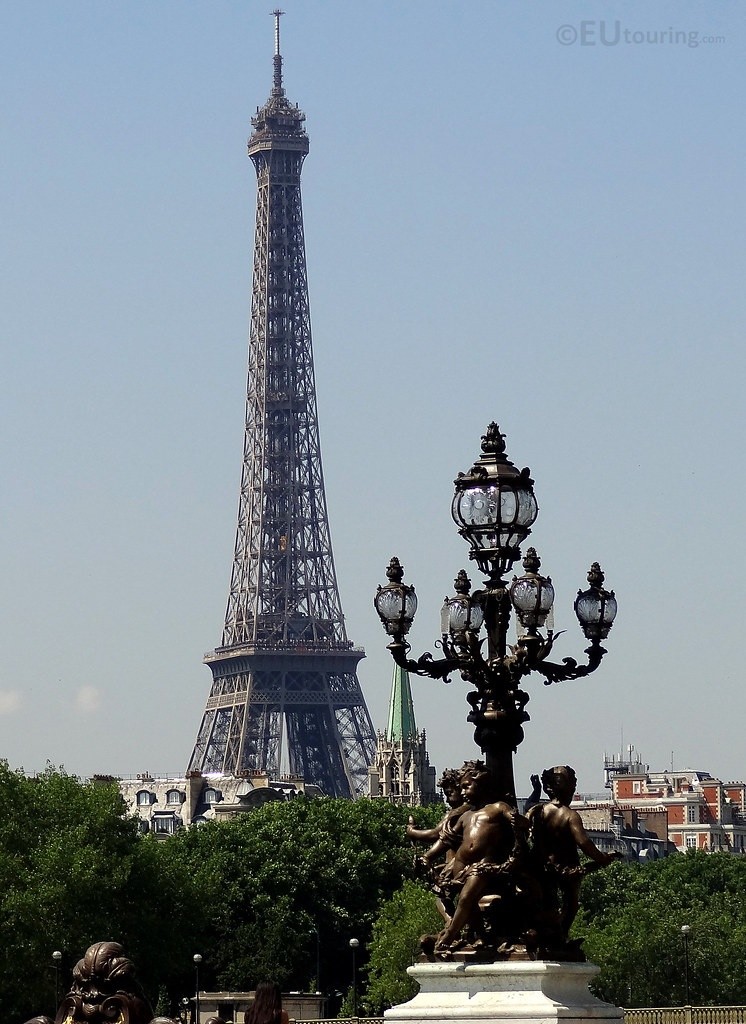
[407,769,471,929]
[522,765,612,947]
[244,980,288,1024]
[414,759,529,951]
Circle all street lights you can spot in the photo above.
[681,925,691,1005]
[193,953,203,1024]
[308,926,320,992]
[349,938,360,1016]
[52,950,62,1014]
